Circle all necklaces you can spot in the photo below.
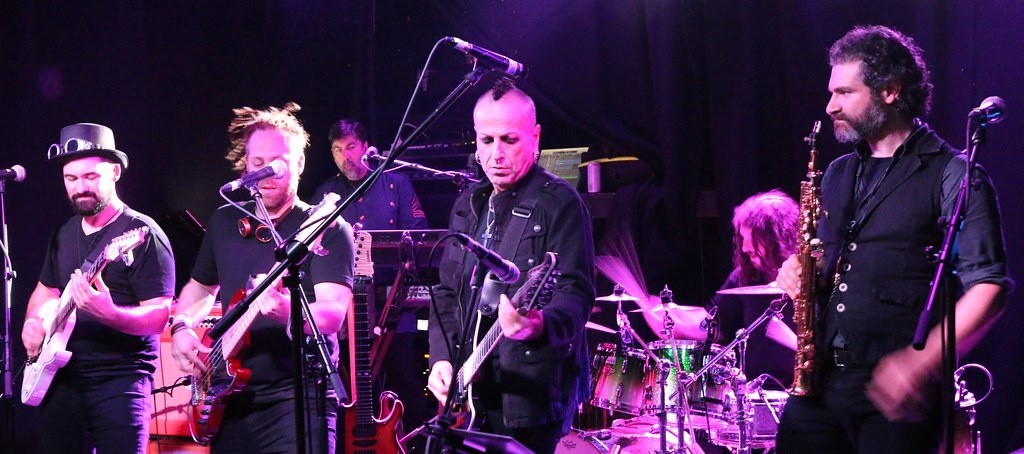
[77,207,122,268]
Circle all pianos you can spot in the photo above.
[364,229,449,386]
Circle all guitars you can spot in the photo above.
[438,250,562,432]
[20,226,150,407]
[189,192,343,446]
[335,230,405,454]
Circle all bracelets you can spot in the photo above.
[173,327,189,334]
[24,319,34,326]
[171,322,186,332]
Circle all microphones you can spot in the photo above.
[221,160,286,191]
[699,306,718,332]
[445,36,524,79]
[456,233,520,283]
[184,375,193,389]
[745,374,768,393]
[954,367,965,382]
[969,96,1009,120]
[0,165,27,182]
[361,146,378,161]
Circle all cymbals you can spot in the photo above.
[585,321,617,334]
[629,303,704,312]
[595,296,640,301]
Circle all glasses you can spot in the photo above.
[48,138,104,159]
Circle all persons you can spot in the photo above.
[313,121,427,282]
[428,77,595,453]
[775,25,1016,454]
[20,123,176,454]
[643,190,800,388]
[172,101,355,454]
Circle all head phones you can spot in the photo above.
[237,197,300,243]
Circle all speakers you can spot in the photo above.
[147,301,223,438]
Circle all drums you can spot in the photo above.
[555,419,706,454]
[640,340,736,429]
[583,342,660,416]
[717,390,790,448]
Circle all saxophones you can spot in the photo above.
[784,120,821,397]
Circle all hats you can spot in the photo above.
[49,122,128,174]
